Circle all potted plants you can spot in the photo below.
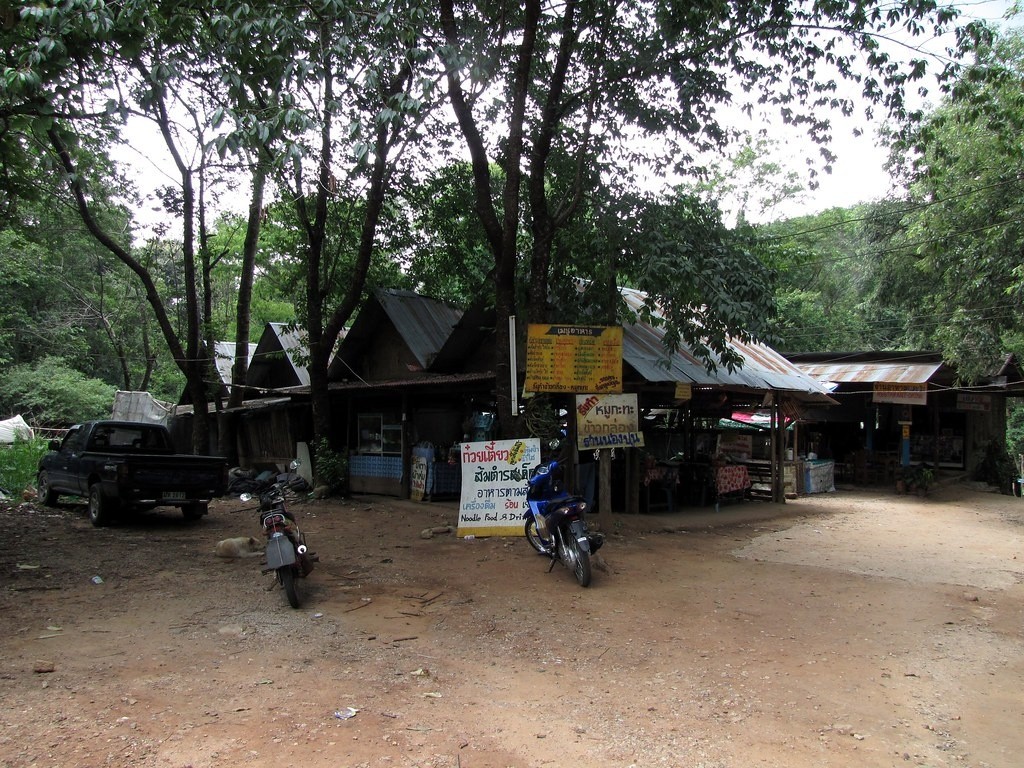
[893,466,937,498]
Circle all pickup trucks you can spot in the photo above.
[38,419,230,529]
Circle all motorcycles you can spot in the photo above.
[240,457,320,609]
[510,437,606,589]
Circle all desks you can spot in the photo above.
[408,461,462,503]
[693,465,751,512]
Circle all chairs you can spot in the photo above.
[92,435,110,450]
[133,439,144,449]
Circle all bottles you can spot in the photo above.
[788,448,793,461]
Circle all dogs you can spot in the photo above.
[213,536,267,558]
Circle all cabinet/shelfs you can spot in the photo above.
[357,413,407,458]
